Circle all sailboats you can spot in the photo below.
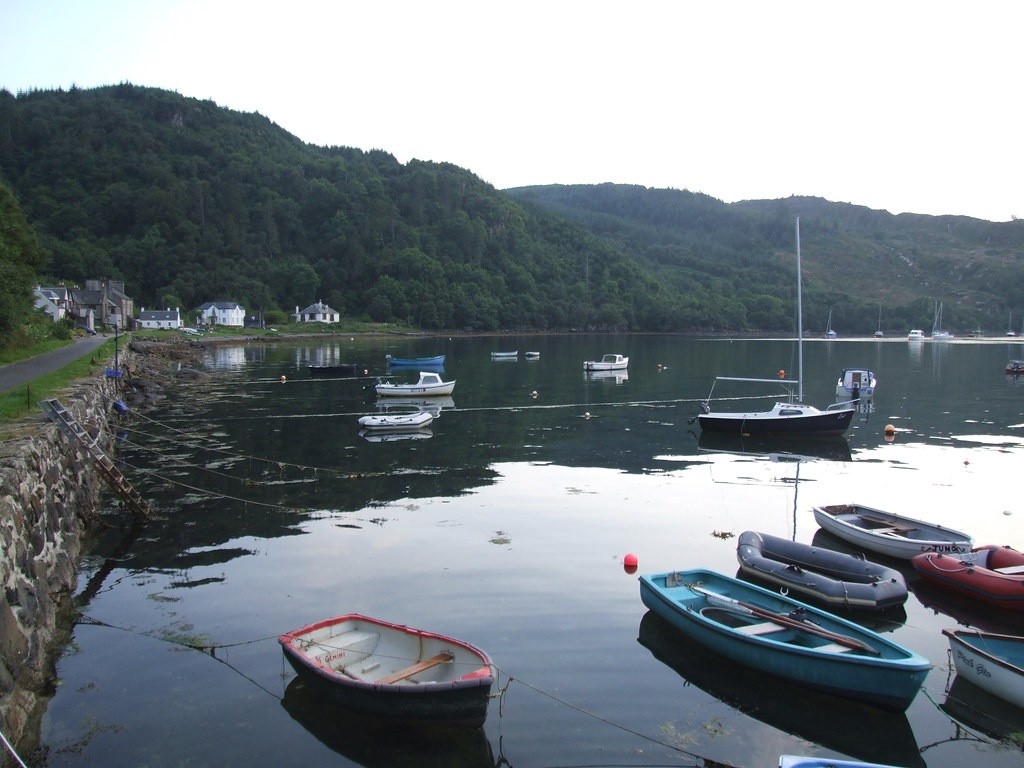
[875,306,884,337]
[1005,311,1017,336]
[696,214,862,437]
[930,300,955,340]
[824,309,836,335]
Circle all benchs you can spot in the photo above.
[809,642,855,654]
[869,525,919,536]
[731,621,796,641]
[376,651,453,681]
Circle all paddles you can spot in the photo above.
[692,585,881,656]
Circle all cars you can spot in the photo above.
[197,326,210,332]
[79,325,96,335]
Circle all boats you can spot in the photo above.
[736,530,909,610]
[943,628,1024,712]
[779,753,892,768]
[583,353,629,372]
[278,613,498,730]
[384,354,446,365]
[836,367,877,397]
[639,567,933,709]
[362,372,458,395]
[524,351,540,356]
[358,413,432,428]
[910,543,1024,613]
[811,503,973,564]
[1005,359,1024,376]
[490,351,517,356]
[908,330,924,342]
[308,364,358,372]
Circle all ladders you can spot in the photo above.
[37,398,156,523]
